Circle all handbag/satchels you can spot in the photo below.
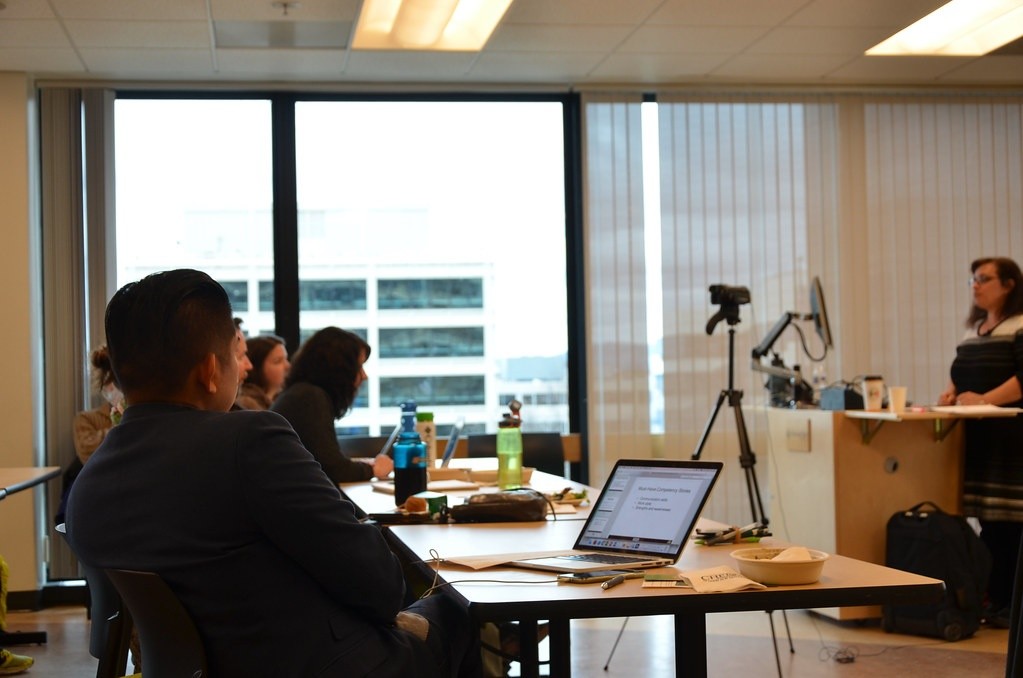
[884,498,983,643]
[449,488,556,524]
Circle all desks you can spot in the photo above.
[338,457,946,678]
[0,466,62,645]
[733,404,1016,622]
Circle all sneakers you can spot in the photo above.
[0,647,34,675]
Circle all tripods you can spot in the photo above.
[603,305,796,678]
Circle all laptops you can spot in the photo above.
[370,423,466,483]
[504,459,724,573]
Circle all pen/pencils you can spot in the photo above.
[601,574,627,591]
[689,517,774,545]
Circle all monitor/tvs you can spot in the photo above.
[808,277,833,347]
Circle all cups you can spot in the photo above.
[888,387,908,412]
[862,375,884,411]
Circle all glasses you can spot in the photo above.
[969,275,1000,285]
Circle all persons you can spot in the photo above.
[64,269,506,678]
[940,258,1023,626]
[233,317,392,481]
[74,347,126,464]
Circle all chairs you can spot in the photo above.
[54,522,209,678]
[468,433,563,473]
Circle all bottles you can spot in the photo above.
[791,365,805,408]
[395,398,436,471]
[496,398,524,490]
[393,433,428,508]
[769,353,785,409]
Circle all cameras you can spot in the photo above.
[710,283,751,305]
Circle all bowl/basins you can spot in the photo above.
[730,547,830,585]
